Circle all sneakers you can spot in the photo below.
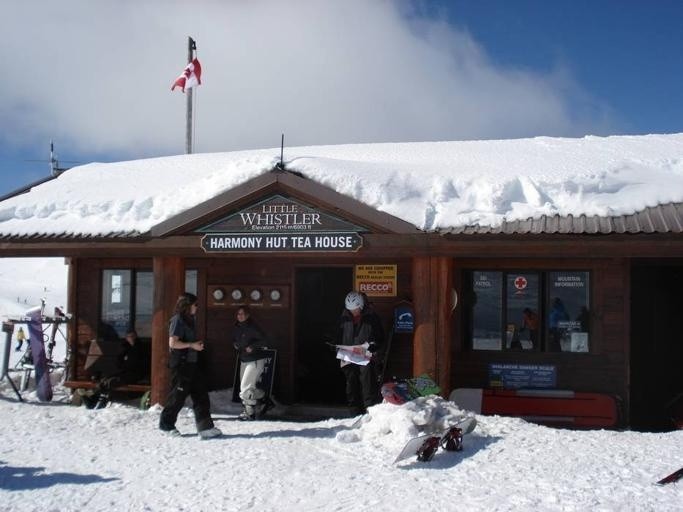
[200,429,222,438]
[83,395,98,408]
[94,394,110,408]
[159,429,181,437]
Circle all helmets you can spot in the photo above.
[345,291,364,310]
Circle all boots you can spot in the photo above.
[259,395,275,414]
[238,406,256,421]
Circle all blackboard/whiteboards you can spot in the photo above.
[231,348,277,404]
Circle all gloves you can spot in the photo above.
[368,342,376,354]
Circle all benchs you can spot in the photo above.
[62,339,151,406]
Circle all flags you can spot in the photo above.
[172,58,202,93]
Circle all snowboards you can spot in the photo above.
[394,417,477,465]
[25,307,54,401]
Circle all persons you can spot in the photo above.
[156,292,222,440]
[232,306,275,422]
[81,329,147,409]
[16,327,26,352]
[519,307,538,348]
[550,298,571,351]
[333,290,379,418]
